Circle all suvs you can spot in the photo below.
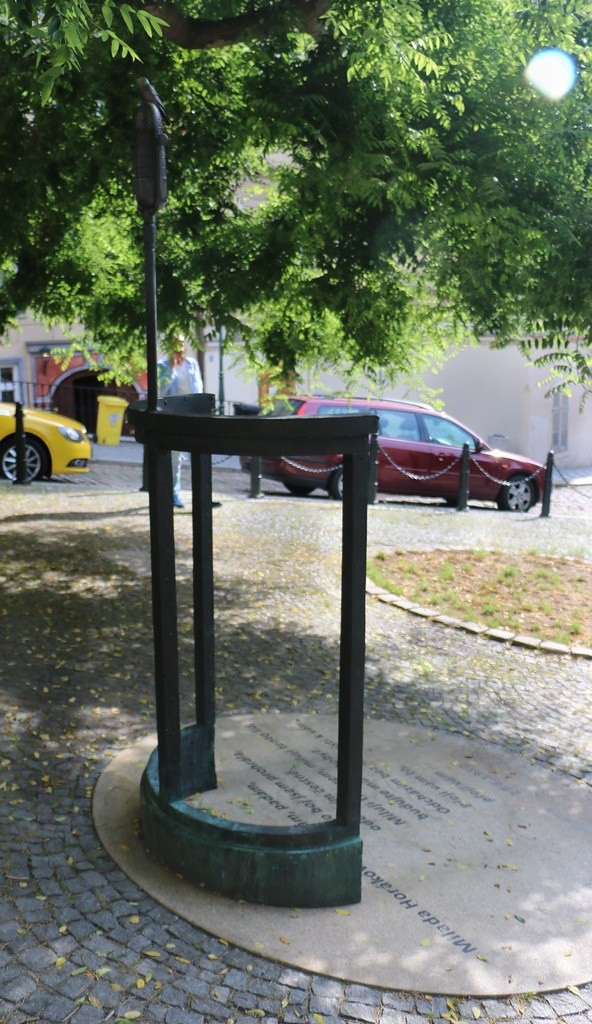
[239,394,547,512]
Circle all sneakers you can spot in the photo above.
[172,494,185,507]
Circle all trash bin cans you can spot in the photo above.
[96,396,129,445]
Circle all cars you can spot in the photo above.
[0,400,93,483]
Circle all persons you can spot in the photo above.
[157,333,204,508]
